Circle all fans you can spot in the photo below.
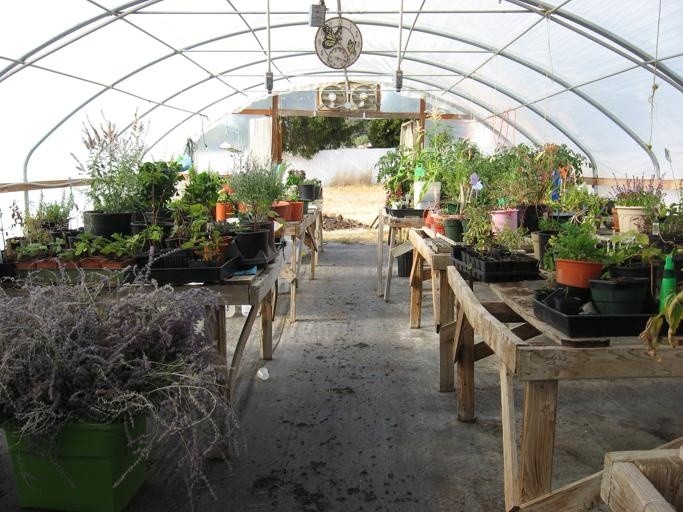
[315,81,381,113]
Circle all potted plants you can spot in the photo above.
[1,104,320,273]
[1,251,244,512]
[373,96,683,338]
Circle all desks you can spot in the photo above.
[443,262,683,511]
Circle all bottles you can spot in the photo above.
[651,214,672,235]
[658,254,678,314]
